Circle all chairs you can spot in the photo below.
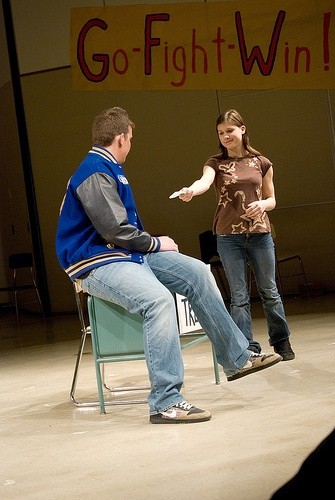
[198,229,309,307]
[0,252,46,317]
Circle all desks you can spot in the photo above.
[88,291,221,416]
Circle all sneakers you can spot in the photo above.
[248,344,260,354]
[274,338,295,361]
[150,400,211,424]
[227,353,283,381]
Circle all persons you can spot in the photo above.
[54,107,283,425]
[178,109,297,360]
